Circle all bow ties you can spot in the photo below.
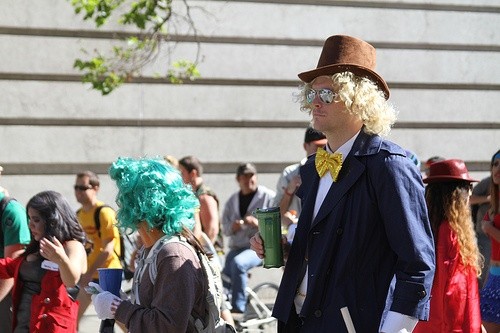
[315,147,343,182]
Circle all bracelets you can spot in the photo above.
[284,189,291,197]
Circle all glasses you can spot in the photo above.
[75,185,93,191]
[305,88,342,105]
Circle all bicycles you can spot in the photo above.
[214,241,280,333]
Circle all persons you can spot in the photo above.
[272,127,329,229]
[249,35,435,333]
[413,158,483,333]
[89,154,237,333]
[68,170,123,333]
[0,191,89,333]
[178,156,225,274]
[218,163,276,311]
[476,150,500,333]
[0,166,31,333]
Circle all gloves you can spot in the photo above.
[119,289,131,303]
[88,281,123,320]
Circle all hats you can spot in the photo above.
[237,163,256,176]
[297,35,390,101]
[304,127,327,143]
[422,159,481,184]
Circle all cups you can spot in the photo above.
[256,207,285,268]
[96,268,124,298]
